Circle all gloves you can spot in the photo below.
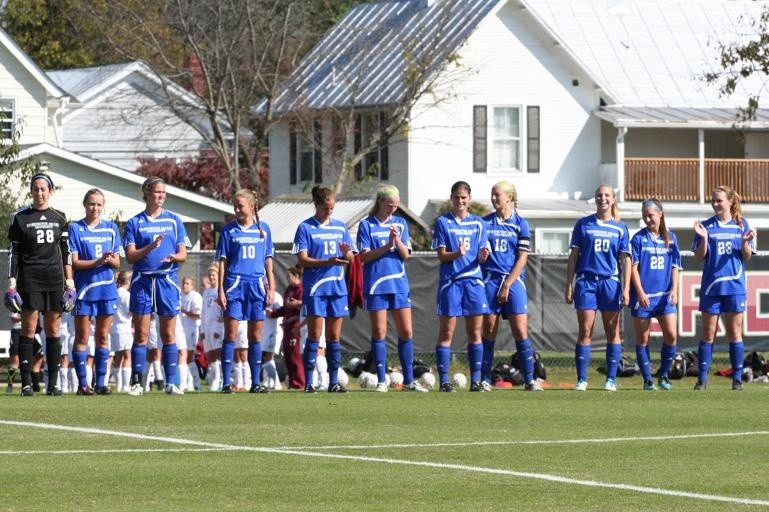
[62,287,76,312]
[4,289,23,313]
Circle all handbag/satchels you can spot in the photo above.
[743,351,768,382]
[492,364,524,385]
[617,353,640,378]
[671,351,699,379]
[512,351,546,380]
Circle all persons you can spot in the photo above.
[432,180,489,392]
[690,184,758,389]
[632,199,682,391]
[356,185,430,392]
[482,182,545,391]
[292,185,360,393]
[565,185,634,391]
[122,176,193,394]
[3,262,336,395]
[68,188,126,394]
[214,188,276,392]
[7,174,76,395]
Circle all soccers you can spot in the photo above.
[420,372,436,389]
[390,372,404,384]
[357,371,376,388]
[348,358,360,372]
[452,373,467,388]
[376,374,390,386]
[337,369,349,388]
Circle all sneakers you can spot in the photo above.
[604,380,615,391]
[21,385,33,396]
[77,385,94,395]
[146,385,153,392]
[659,378,672,389]
[328,384,348,392]
[115,387,123,393]
[31,384,40,392]
[268,381,273,388]
[693,380,707,389]
[130,384,143,396]
[525,380,544,391]
[47,387,62,395]
[165,383,183,394]
[231,385,240,392]
[733,381,742,389]
[576,380,587,392]
[470,383,486,392]
[402,381,428,392]
[222,386,234,394]
[186,384,194,392]
[305,383,316,392]
[375,383,388,392]
[239,387,250,393]
[274,382,282,390]
[249,385,268,393]
[95,386,112,395]
[157,383,164,391]
[6,384,13,392]
[123,386,131,394]
[69,387,78,394]
[643,382,656,391]
[440,383,455,392]
[209,384,221,391]
[193,384,201,390]
[61,388,69,394]
[479,381,491,392]
